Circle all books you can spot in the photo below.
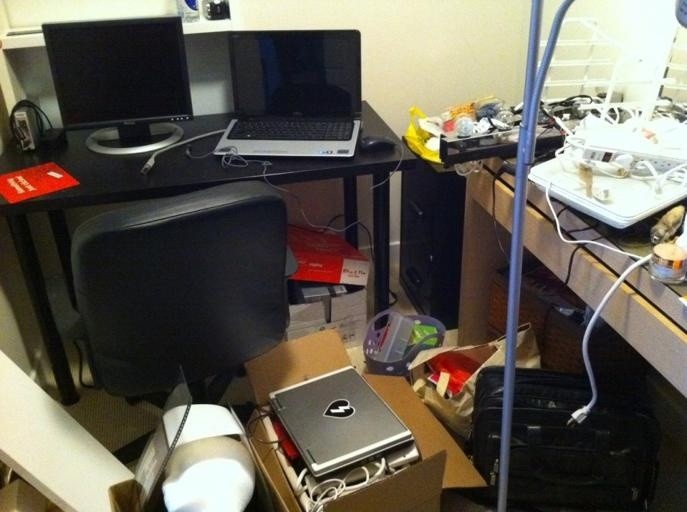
[0,161,80,206]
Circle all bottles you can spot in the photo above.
[177,0,200,22]
[454,113,473,137]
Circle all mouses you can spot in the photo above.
[358,135,394,152]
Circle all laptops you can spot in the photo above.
[267,365,414,480]
[211,29,364,157]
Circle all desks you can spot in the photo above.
[0,101,414,404]
[457,151,687,402]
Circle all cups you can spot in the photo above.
[205,0,227,20]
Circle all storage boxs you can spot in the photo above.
[236,328,489,511]
[283,218,373,347]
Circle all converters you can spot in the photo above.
[40,128,65,146]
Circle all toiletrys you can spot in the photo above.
[647,242,687,282]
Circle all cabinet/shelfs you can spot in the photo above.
[0,1,241,50]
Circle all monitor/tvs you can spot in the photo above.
[40,15,194,154]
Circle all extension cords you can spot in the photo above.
[580,145,687,183]
[303,442,420,499]
